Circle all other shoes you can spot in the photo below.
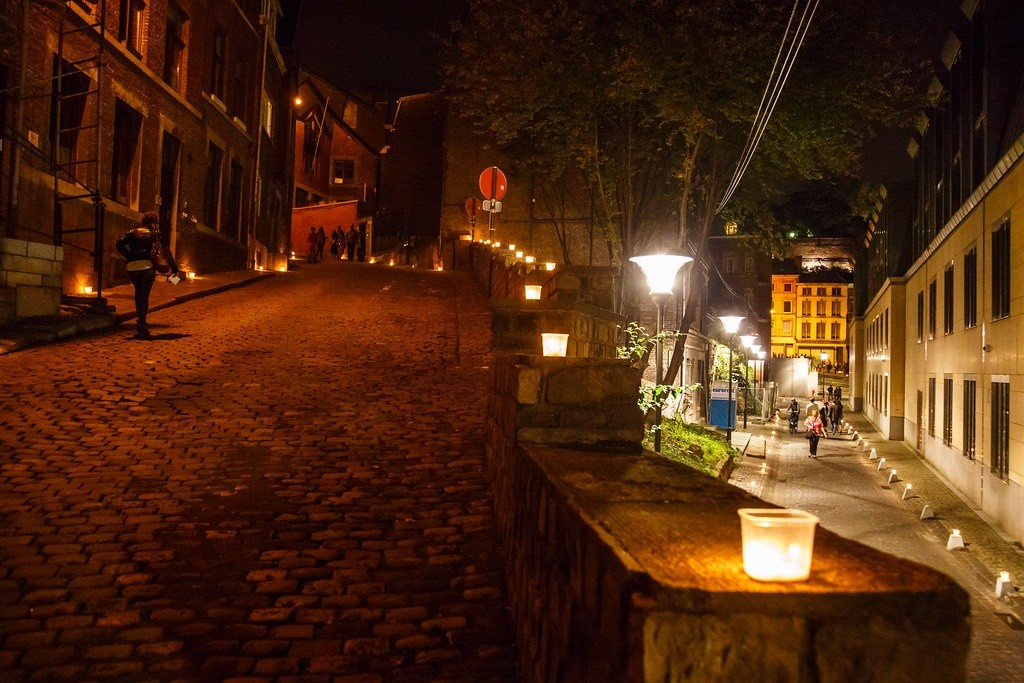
[809,454,817,458]
[135,319,151,339]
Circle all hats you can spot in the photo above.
[790,398,796,401]
[809,397,816,401]
[349,223,355,229]
[824,401,828,405]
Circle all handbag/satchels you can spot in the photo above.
[803,428,814,440]
[149,229,170,273]
[330,244,338,254]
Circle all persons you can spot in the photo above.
[786,397,800,427]
[806,397,843,436]
[307,223,366,263]
[804,409,827,459]
[827,385,842,401]
[116,212,178,340]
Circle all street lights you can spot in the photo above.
[719,315,745,448]
[740,334,767,428]
[628,253,694,452]
[820,351,829,401]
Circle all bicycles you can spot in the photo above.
[787,411,799,435]
